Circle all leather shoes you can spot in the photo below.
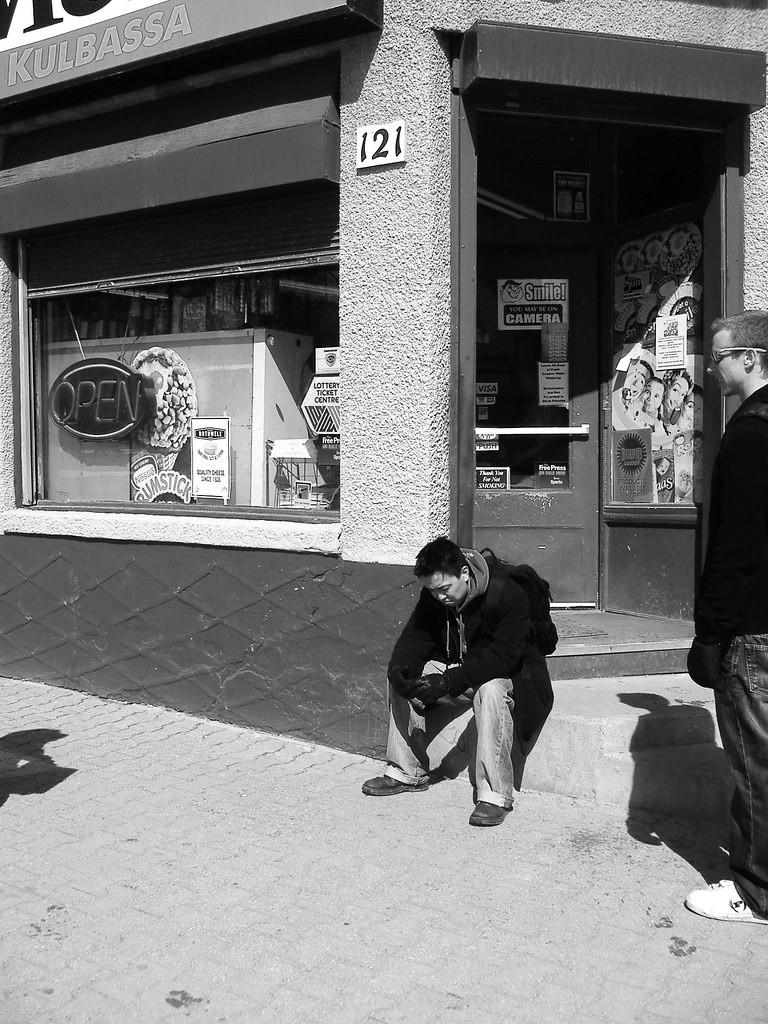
[470,801,513,825]
[362,775,429,795]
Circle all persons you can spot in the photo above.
[686,310,768,925]
[361,536,552,827]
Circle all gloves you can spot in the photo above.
[687,639,722,688]
[411,673,450,705]
[388,659,420,699]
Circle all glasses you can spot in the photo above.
[711,346,767,364]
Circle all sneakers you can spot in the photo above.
[685,879,768,924]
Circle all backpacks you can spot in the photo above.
[480,547,558,656]
